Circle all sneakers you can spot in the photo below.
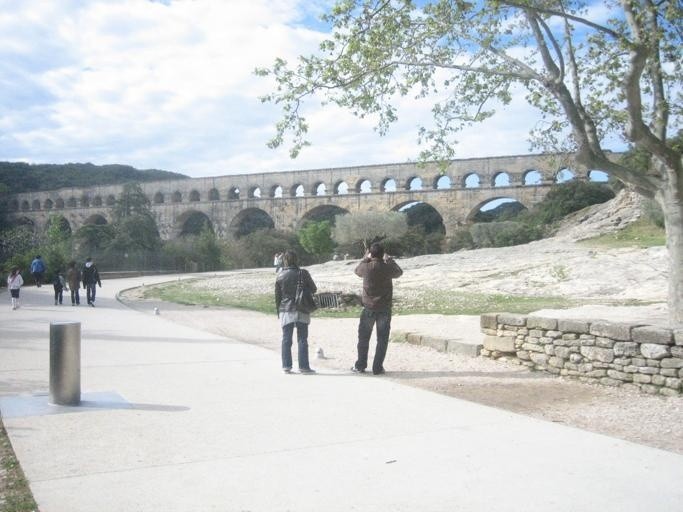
[55,299,95,307]
[299,369,315,374]
[283,367,291,374]
[352,366,365,372]
[373,367,385,374]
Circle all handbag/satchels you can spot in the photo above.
[296,285,316,313]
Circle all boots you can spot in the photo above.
[12,298,19,309]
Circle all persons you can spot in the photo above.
[51,268,67,306]
[274,252,288,273]
[273,251,317,375]
[7,265,23,311]
[80,258,101,307]
[66,261,81,306]
[352,243,402,374]
[30,254,45,287]
